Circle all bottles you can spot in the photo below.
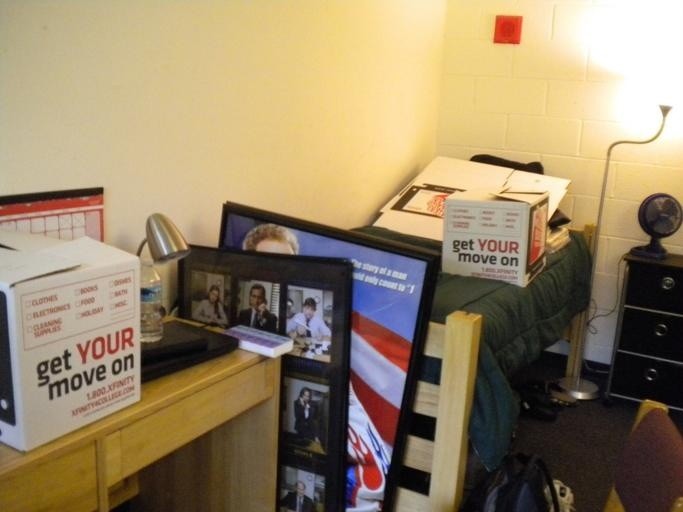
[140,258,165,344]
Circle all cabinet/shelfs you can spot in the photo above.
[601,253,683,412]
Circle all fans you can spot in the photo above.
[630,192,683,260]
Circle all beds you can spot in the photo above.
[347,221,595,511]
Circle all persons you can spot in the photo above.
[279,479,319,512]
[236,283,279,334]
[292,386,323,444]
[284,298,301,323]
[238,222,395,511]
[187,283,231,332]
[285,297,333,348]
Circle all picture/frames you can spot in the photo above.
[218,200,442,509]
[176,243,353,512]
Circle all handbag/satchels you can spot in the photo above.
[461,449,558,512]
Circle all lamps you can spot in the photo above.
[136,213,192,264]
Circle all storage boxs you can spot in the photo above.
[442,188,549,289]
[0,229,142,455]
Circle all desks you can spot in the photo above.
[1,313,281,511]
